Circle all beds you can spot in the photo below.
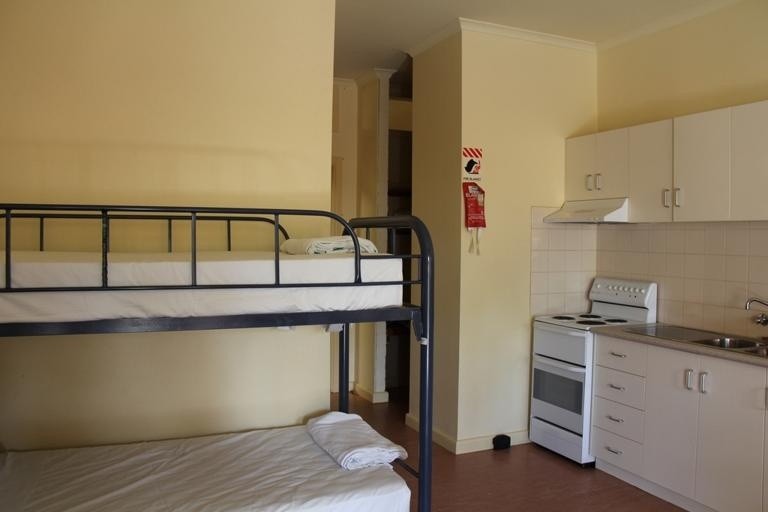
[0,204,435,512]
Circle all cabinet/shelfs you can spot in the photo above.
[641,344,768,512]
[589,333,647,488]
[730,101,768,221]
[564,128,628,202]
[627,108,730,223]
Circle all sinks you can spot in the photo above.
[745,348,768,359]
[692,338,756,348]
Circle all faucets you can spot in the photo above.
[746,297,768,310]
